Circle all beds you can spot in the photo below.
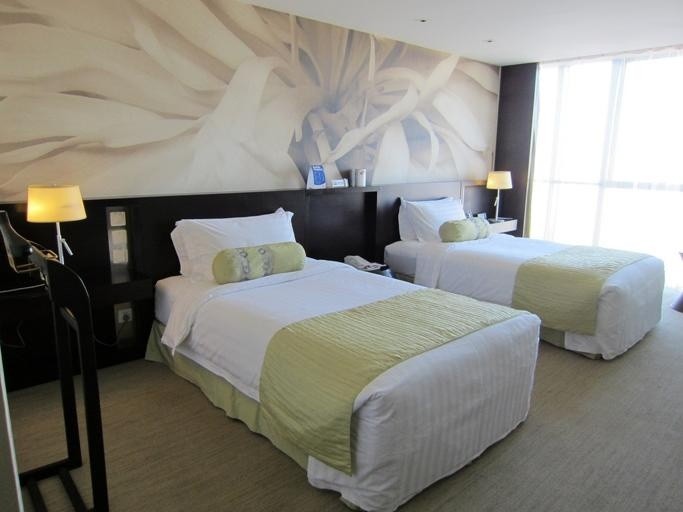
[140,245,543,510]
[379,223,669,368]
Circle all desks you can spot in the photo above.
[478,214,518,236]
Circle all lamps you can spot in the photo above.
[22,179,86,268]
[484,168,513,223]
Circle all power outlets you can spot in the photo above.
[116,308,132,325]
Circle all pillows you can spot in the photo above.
[406,196,467,243]
[395,194,455,244]
[163,205,309,289]
[437,216,491,244]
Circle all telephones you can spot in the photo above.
[344,255,372,268]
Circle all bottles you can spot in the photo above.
[349,169,356,187]
[356,169,367,188]
